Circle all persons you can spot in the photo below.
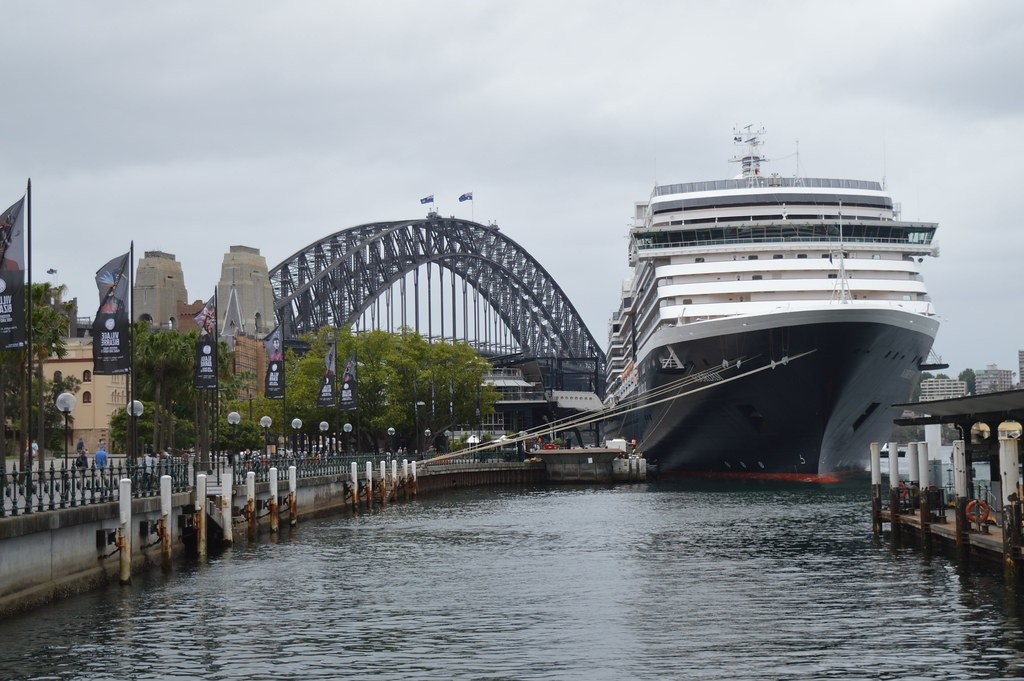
[77,438,83,452]
[97,439,105,447]
[245,448,252,468]
[228,450,233,467]
[76,451,88,479]
[141,447,156,497]
[158,449,171,475]
[95,447,109,480]
[32,440,38,451]
[398,447,407,454]
[910,481,919,497]
[181,450,190,459]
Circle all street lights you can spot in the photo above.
[319,421,329,457]
[292,419,303,457]
[344,423,352,456]
[227,411,241,485]
[444,430,451,453]
[127,400,145,497]
[387,427,395,450]
[260,415,272,482]
[56,393,76,504]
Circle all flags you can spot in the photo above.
[92,252,131,375]
[0,196,28,351]
[317,344,335,407]
[420,195,433,204]
[459,192,472,201]
[192,295,216,389]
[263,323,284,399]
[340,353,357,410]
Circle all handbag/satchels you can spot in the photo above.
[76,458,82,466]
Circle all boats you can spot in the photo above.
[880,444,905,458]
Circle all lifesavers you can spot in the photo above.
[966,499,989,522]
[899,480,917,499]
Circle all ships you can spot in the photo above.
[602,122,950,483]
